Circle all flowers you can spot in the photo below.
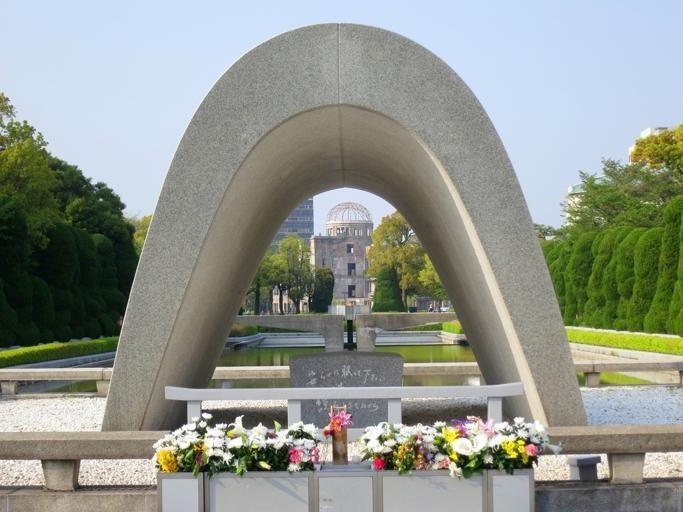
[151,412,327,480]
[322,411,352,437]
[358,417,550,481]
